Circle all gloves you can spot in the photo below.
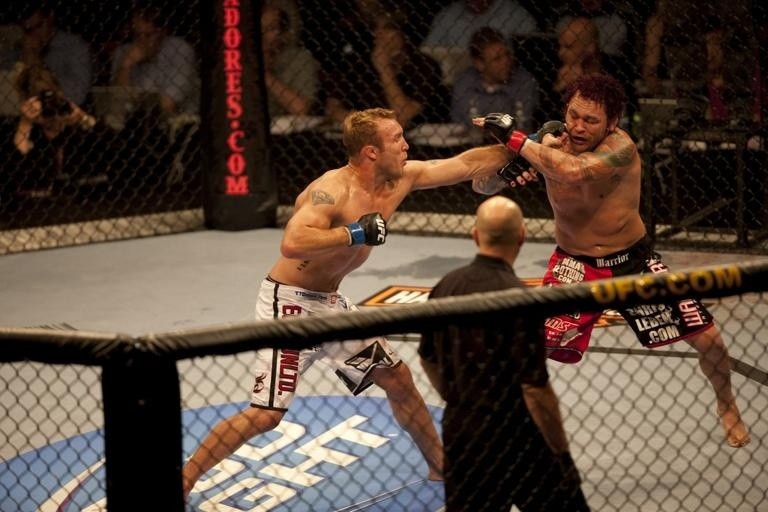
[552,452,581,497]
[345,213,388,248]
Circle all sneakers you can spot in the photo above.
[720,118,753,139]
[665,108,705,132]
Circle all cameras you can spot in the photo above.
[39,88,74,118]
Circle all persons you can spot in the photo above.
[180,108,569,504]
[417,194,593,511]
[472,74,751,448]
